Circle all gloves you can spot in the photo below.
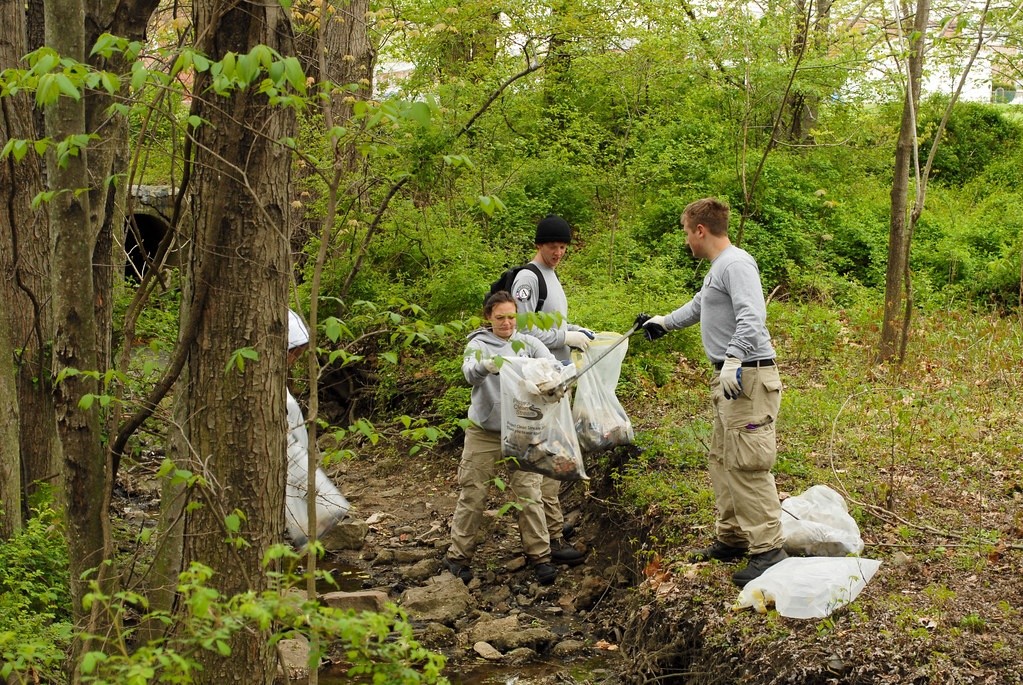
[571,325,596,342]
[642,314,668,340]
[484,353,501,375]
[718,355,744,400]
[565,330,591,352]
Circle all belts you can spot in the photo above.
[714,357,774,370]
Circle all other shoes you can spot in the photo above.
[534,562,557,584]
[550,542,586,566]
[562,524,576,540]
[442,554,474,583]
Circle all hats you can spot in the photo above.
[534,215,571,245]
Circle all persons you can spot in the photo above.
[285,308,311,451]
[442,289,566,582]
[508,216,596,566]
[642,198,790,588]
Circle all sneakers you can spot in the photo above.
[732,547,789,583]
[688,539,743,564]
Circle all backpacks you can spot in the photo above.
[484,262,548,312]
